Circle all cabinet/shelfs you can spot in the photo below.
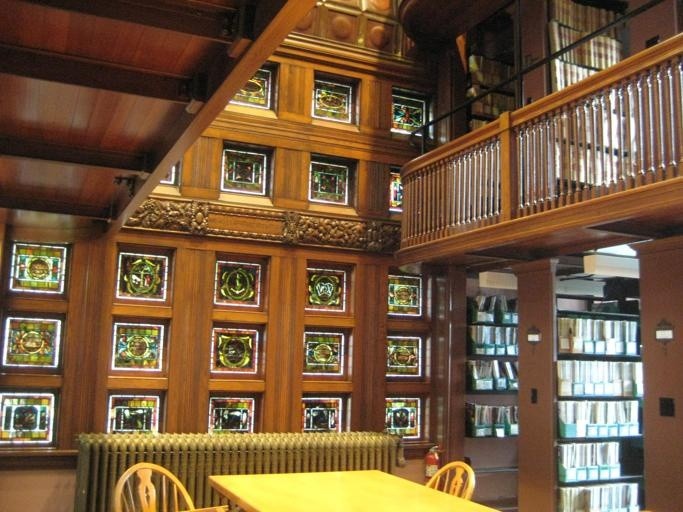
[466,53,516,121]
[467,317,520,438]
[547,1,627,155]
[558,310,643,489]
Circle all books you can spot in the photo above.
[466,294,644,512]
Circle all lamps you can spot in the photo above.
[583,252,639,279]
[477,269,517,290]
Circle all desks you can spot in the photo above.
[208,468,504,512]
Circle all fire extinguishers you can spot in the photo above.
[425,446,444,488]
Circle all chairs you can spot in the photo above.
[423,459,477,500]
[109,460,230,512]
